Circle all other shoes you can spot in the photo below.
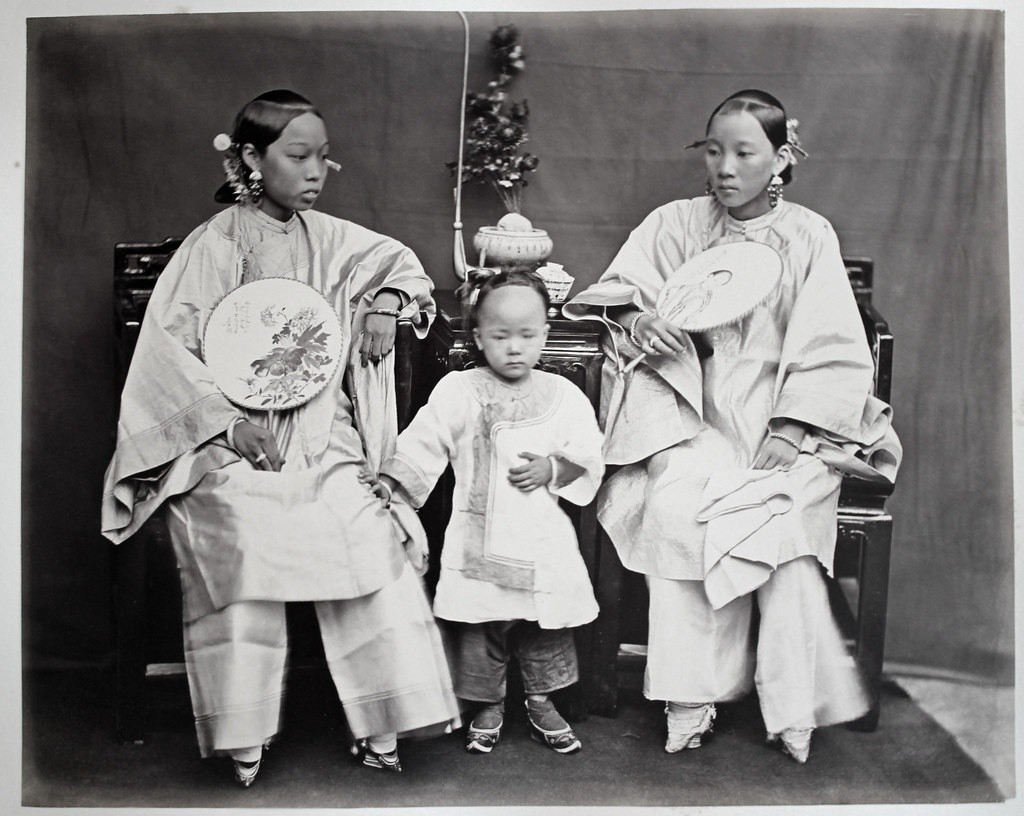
[525,699,583,755]
[780,727,815,763]
[234,764,260,788]
[466,721,503,754]
[664,702,716,753]
[364,748,402,773]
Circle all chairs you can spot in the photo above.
[109,237,414,748]
[586,256,892,732]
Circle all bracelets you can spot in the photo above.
[379,480,393,506]
[628,312,657,348]
[227,415,250,449]
[363,308,403,319]
[770,433,801,454]
[548,454,559,486]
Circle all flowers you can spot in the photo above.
[444,25,540,212]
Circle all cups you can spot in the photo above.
[532,261,574,305]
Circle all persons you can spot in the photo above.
[358,269,605,753]
[561,88,902,764]
[102,90,462,788]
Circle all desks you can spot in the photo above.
[422,287,603,717]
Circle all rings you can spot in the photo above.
[254,452,267,463]
[649,336,661,347]
